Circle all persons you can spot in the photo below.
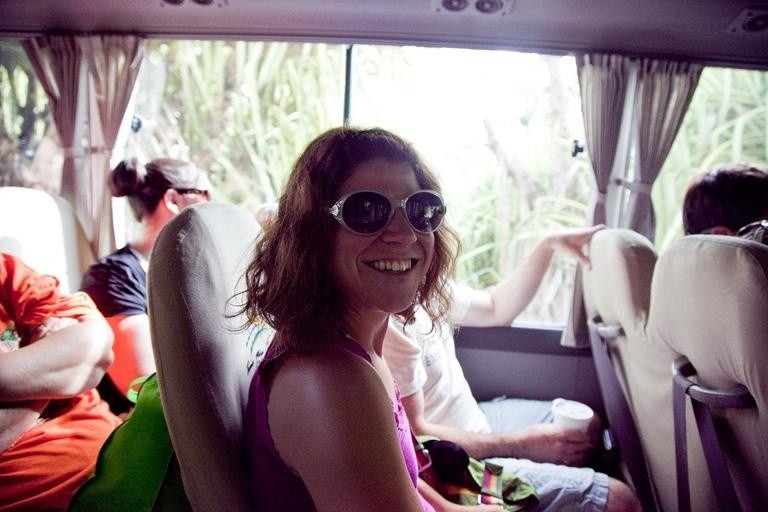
[682,165,768,235]
[220,129,504,511]
[1,254,193,510]
[380,223,641,511]
[76,158,213,415]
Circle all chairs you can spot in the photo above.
[148,202,280,510]
[579,227,768,512]
[1,187,85,299]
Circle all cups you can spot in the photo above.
[552,399,594,433]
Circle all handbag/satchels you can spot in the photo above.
[417,432,539,510]
[67,373,182,510]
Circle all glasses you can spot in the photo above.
[175,187,211,202]
[324,188,448,235]
